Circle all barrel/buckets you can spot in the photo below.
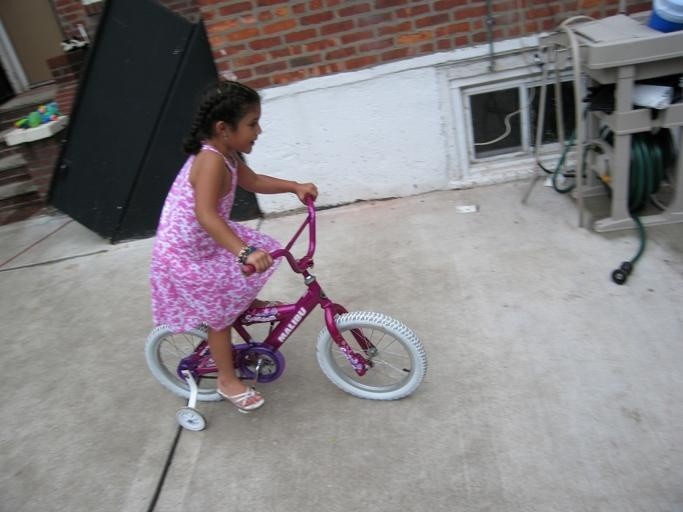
[265,300,289,307]
[216,386,265,411]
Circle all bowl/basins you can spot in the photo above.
[215,144,235,165]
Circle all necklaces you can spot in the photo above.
[215,144,235,165]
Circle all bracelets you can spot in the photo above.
[237,244,256,264]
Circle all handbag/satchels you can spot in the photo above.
[237,244,256,264]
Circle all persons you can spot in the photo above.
[149,81,318,411]
